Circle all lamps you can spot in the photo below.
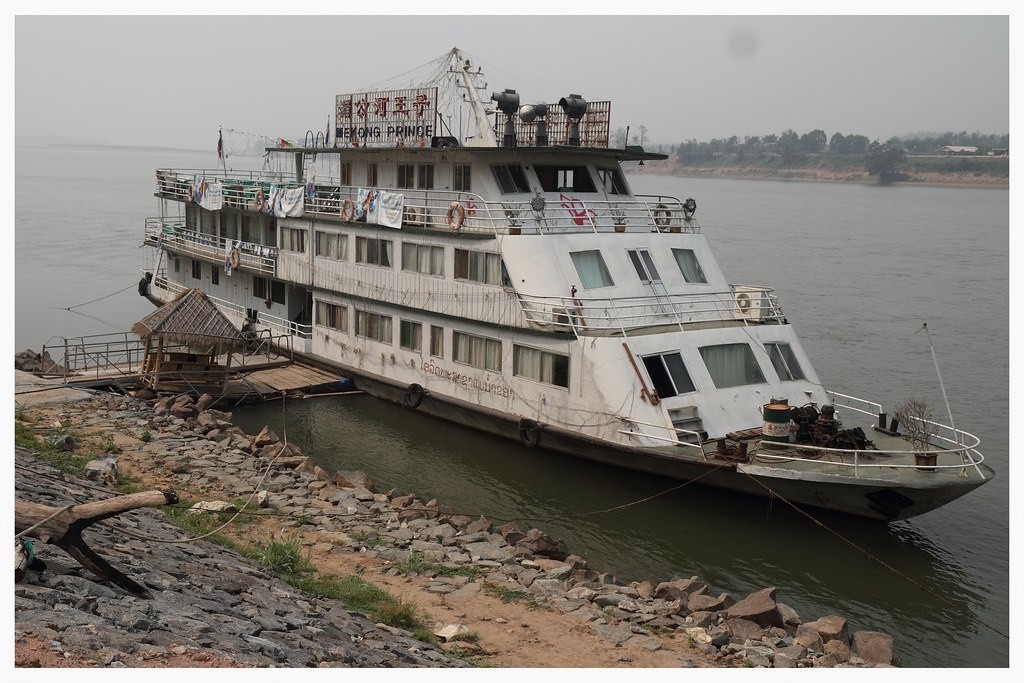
[559,94,587,146]
[519,104,548,146]
[491,89,519,146]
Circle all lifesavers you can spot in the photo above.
[446,201,464,230]
[403,383,425,408]
[340,199,353,221]
[254,191,264,212]
[227,249,239,269]
[241,324,256,344]
[654,204,672,230]
[138,278,147,296]
[518,419,540,447]
[188,183,195,201]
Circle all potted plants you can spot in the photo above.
[500,199,522,235]
[895,394,943,471]
[609,205,626,232]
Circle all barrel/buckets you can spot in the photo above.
[762,404,790,448]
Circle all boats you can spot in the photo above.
[134,47,997,522]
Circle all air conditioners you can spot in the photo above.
[734,286,769,322]
[552,308,579,333]
